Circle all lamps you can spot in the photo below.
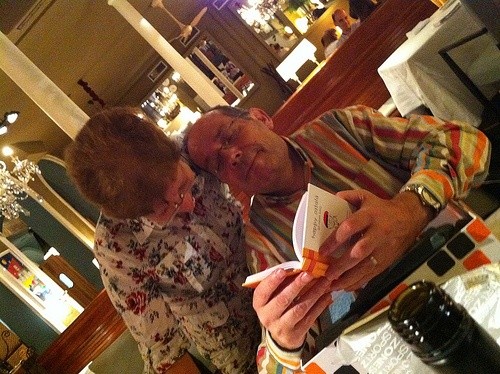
[0,111,21,136]
[276,38,327,93]
[0,146,42,220]
[27,228,62,261]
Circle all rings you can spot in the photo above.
[371,257,378,267]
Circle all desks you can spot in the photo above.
[377,0,500,131]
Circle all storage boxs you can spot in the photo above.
[297,197,500,374]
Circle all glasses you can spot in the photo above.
[210,113,249,173]
[135,154,201,231]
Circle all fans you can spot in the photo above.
[150,0,208,43]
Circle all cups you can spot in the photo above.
[388,280,500,374]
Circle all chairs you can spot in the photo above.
[437,0,499,184]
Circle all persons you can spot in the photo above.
[187,107,490,374]
[319,1,379,59]
[63,107,246,374]
[214,9,328,81]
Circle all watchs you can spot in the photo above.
[402,184,441,221]
